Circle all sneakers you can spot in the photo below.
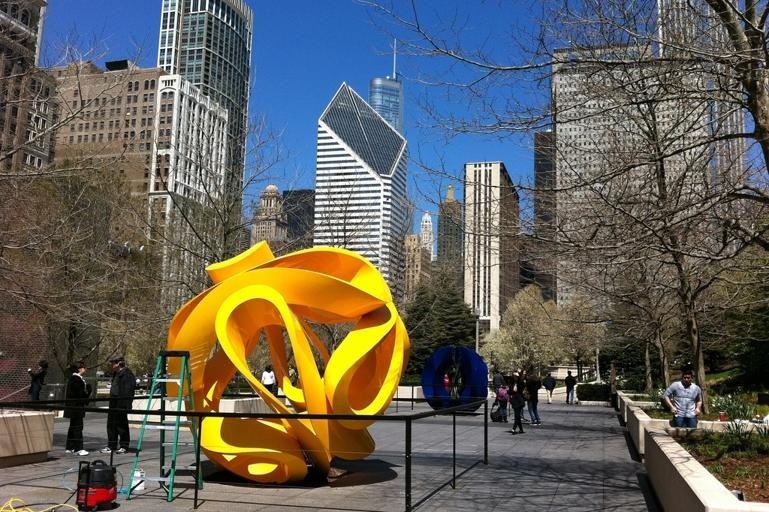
[116,448,127,454]
[65,449,76,453]
[101,446,112,454]
[531,421,542,425]
[74,449,88,455]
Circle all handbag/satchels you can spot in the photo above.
[491,403,502,421]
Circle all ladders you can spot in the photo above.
[125,351,203,502]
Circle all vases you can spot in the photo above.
[719,412,727,421]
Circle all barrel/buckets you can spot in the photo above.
[132,470,146,490]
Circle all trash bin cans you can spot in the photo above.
[47,384,65,410]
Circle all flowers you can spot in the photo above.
[712,394,733,412]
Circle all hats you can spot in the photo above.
[110,354,125,362]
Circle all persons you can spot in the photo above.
[565,371,576,404]
[287,364,297,387]
[26,360,49,401]
[490,365,542,435]
[100,354,136,453]
[261,364,276,396]
[61,361,93,456]
[541,371,557,405]
[662,368,703,428]
